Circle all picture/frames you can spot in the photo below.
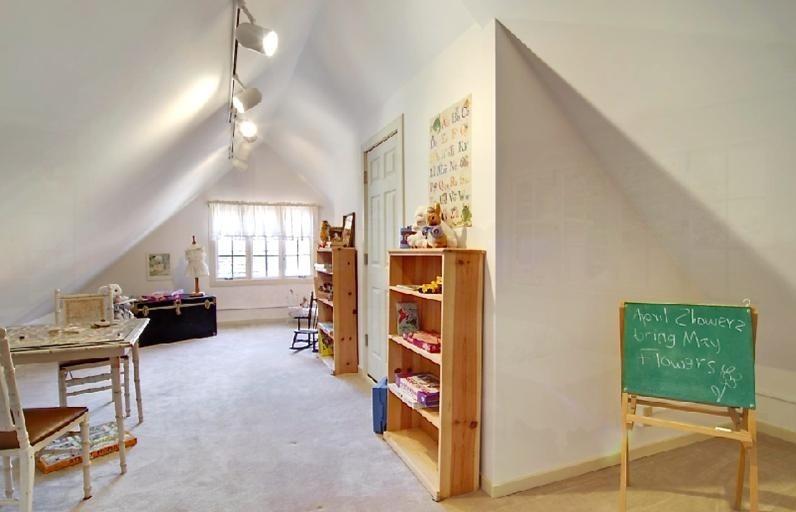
[146,252,173,280]
[338,211,356,247]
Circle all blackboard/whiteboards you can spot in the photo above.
[621,302,755,412]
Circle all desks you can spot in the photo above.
[0,318,149,474]
[133,295,217,347]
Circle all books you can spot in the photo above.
[396,372,440,405]
[397,302,419,335]
[329,228,345,246]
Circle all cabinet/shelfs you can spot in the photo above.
[383,247,486,502]
[314,247,358,375]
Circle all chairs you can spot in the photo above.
[0,328,93,512]
[56,287,132,417]
[290,292,319,353]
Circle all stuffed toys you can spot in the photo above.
[97,284,136,320]
[424,204,447,247]
[407,207,457,248]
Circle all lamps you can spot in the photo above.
[235,0,278,57]
[184,233,209,297]
[233,72,262,114]
